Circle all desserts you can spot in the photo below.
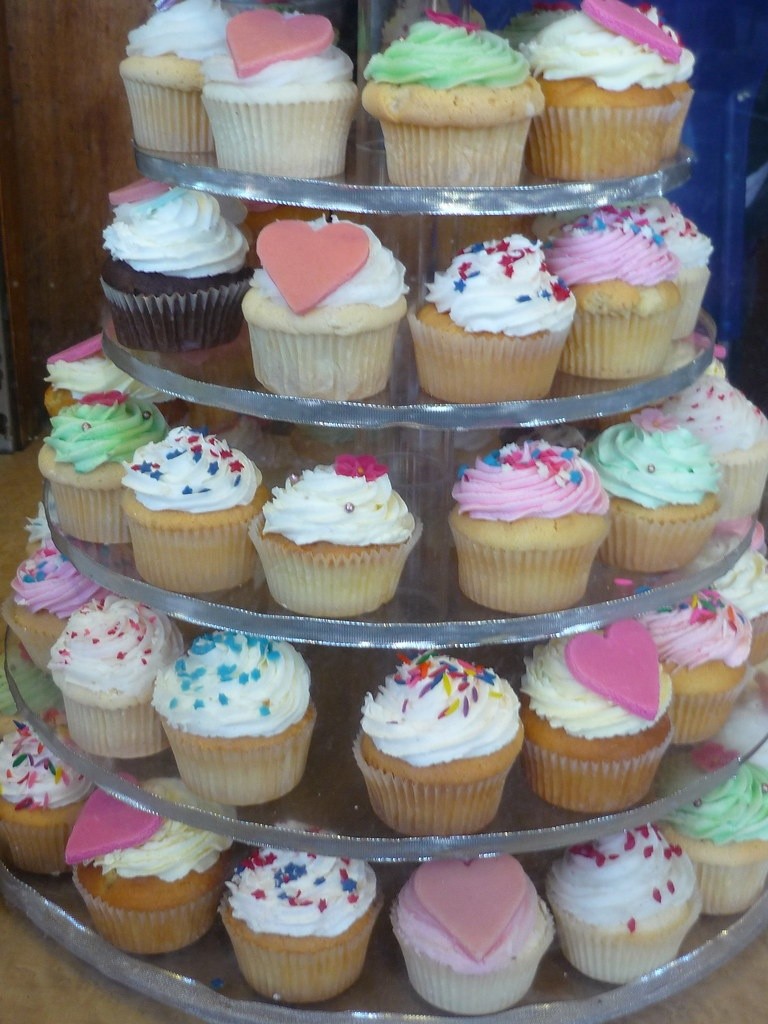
[0,0,768,1014]
